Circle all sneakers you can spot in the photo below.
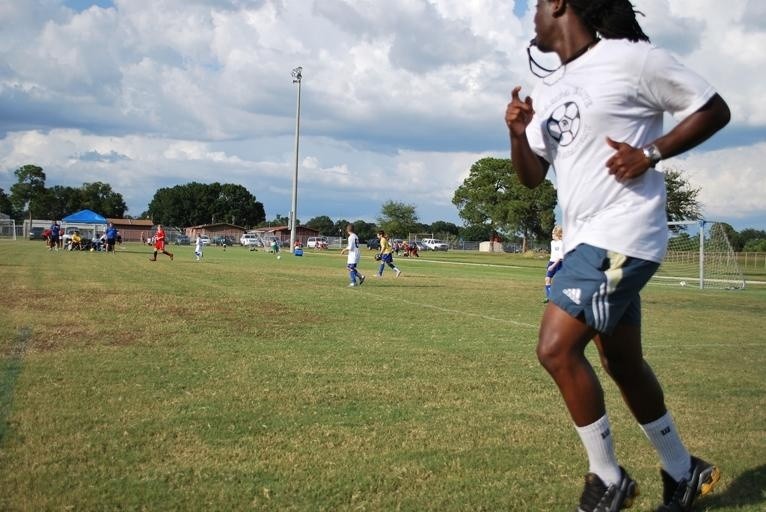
[541,298,549,303]
[350,271,401,286]
[576,466,640,512]
[656,456,720,512]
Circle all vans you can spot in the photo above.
[307,237,329,250]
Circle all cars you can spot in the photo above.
[147,233,237,246]
[265,235,305,248]
[366,236,427,254]
[29,226,45,242]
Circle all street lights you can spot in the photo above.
[289,66,303,254]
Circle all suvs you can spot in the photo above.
[240,233,259,247]
[421,238,449,253]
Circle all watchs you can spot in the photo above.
[642,145,662,171]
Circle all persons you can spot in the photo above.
[195,233,202,260]
[295,239,300,248]
[271,240,277,254]
[373,231,401,278]
[141,232,145,244]
[221,235,227,251]
[389,240,419,258]
[498,0,731,512]
[49,221,126,255]
[149,224,173,261]
[315,240,319,249]
[340,225,365,287]
[541,225,564,302]
